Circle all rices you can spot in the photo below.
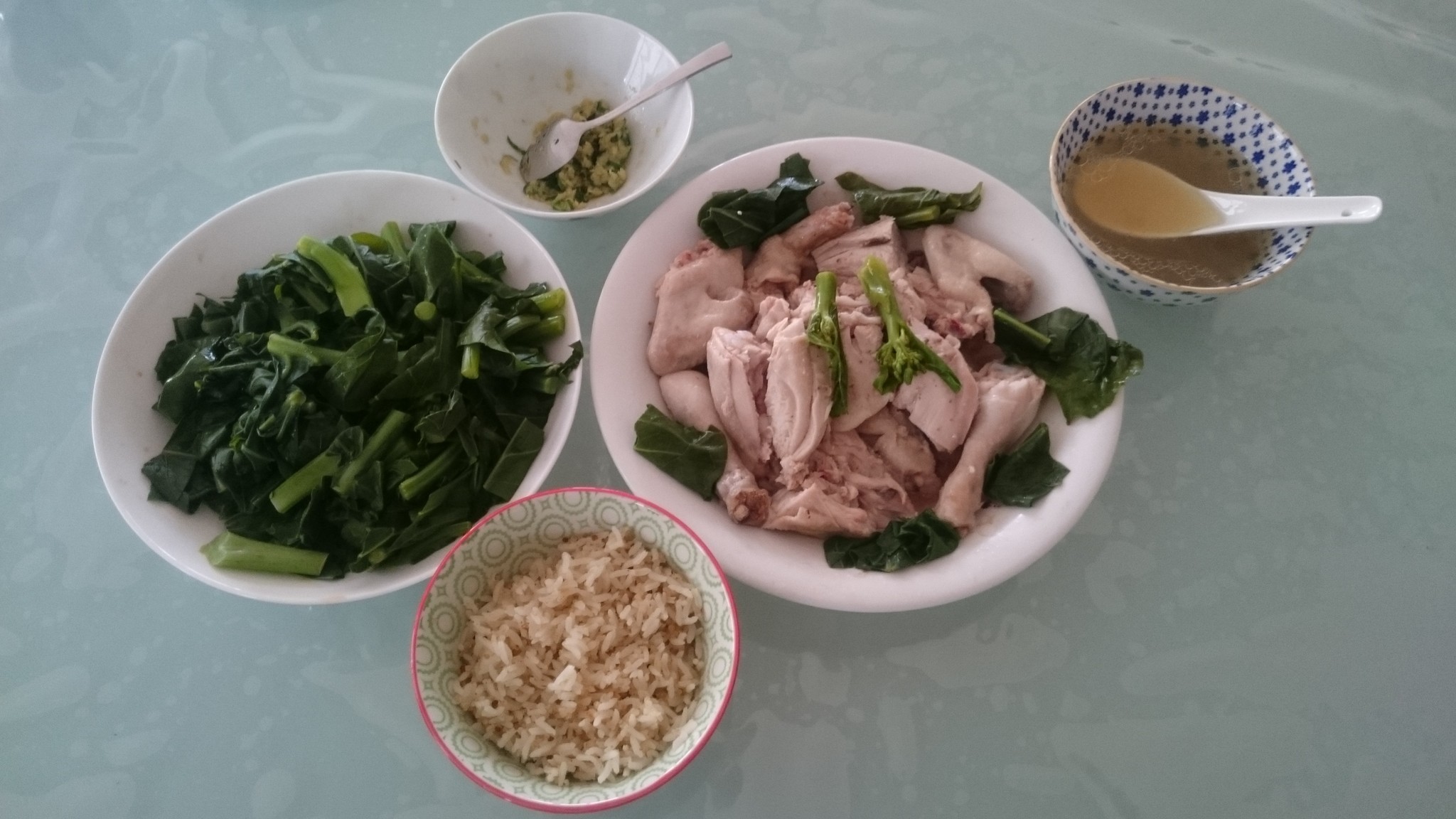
[452,521,709,791]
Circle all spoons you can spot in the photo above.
[521,40,732,183]
[1072,155,1384,238]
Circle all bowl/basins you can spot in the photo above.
[1049,78,1312,308]
[412,486,742,812]
[436,11,699,217]
[89,170,581,610]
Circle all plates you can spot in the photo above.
[591,137,1127,615]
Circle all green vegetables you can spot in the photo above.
[137,216,587,577]
